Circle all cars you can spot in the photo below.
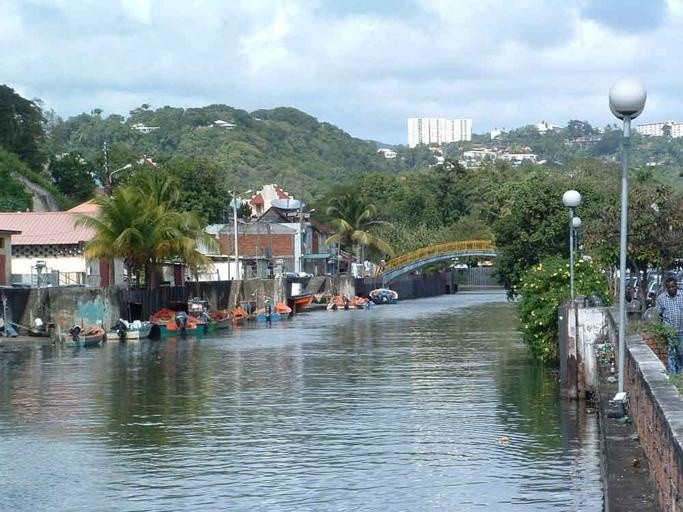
[626,267,683,304]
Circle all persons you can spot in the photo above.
[656,278,682,373]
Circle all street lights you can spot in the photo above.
[109,164,132,184]
[563,190,582,300]
[608,77,647,397]
[227,188,253,280]
